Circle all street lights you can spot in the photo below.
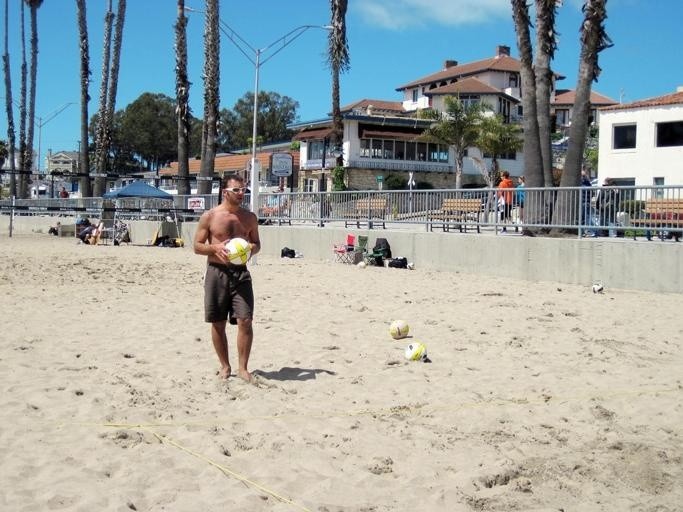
[183,4,335,226]
[0,95,80,199]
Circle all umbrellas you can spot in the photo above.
[102,180,176,221]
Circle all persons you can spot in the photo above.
[48,222,60,236]
[515,175,524,221]
[191,175,260,385]
[58,187,68,197]
[581,171,592,222]
[258,191,277,213]
[269,187,286,213]
[495,170,518,233]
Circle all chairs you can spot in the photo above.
[332,233,388,267]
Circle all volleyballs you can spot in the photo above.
[224,238,251,266]
[593,284,602,294]
[407,262,415,269]
[389,321,408,339]
[357,261,365,268]
[406,342,427,363]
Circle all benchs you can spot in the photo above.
[427,198,483,233]
[344,197,389,230]
[630,197,683,242]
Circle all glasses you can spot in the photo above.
[224,187,246,193]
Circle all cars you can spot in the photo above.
[458,176,600,211]
